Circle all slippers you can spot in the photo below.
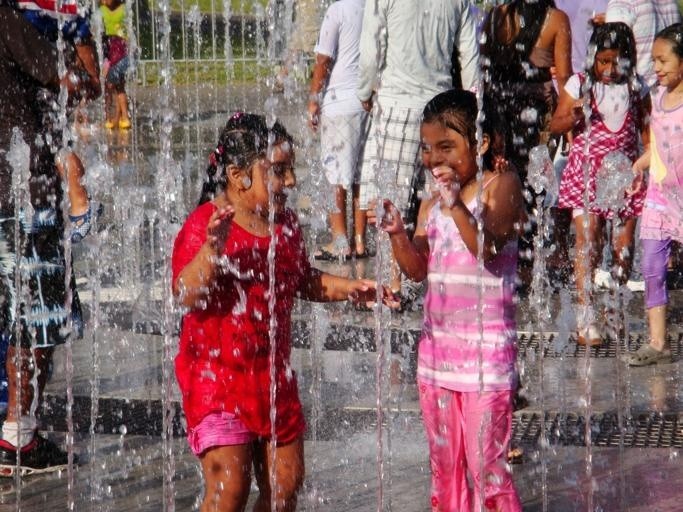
[315,247,352,261]
[353,248,367,259]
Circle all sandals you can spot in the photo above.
[627,341,672,366]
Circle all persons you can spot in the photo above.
[172,112,394,512]
[273,1,683,512]
[0,2,134,475]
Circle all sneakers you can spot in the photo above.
[625,279,644,291]
[0,432,80,477]
[579,326,602,347]
[592,268,620,291]
[68,202,104,244]
[106,118,113,129]
[119,117,131,129]
